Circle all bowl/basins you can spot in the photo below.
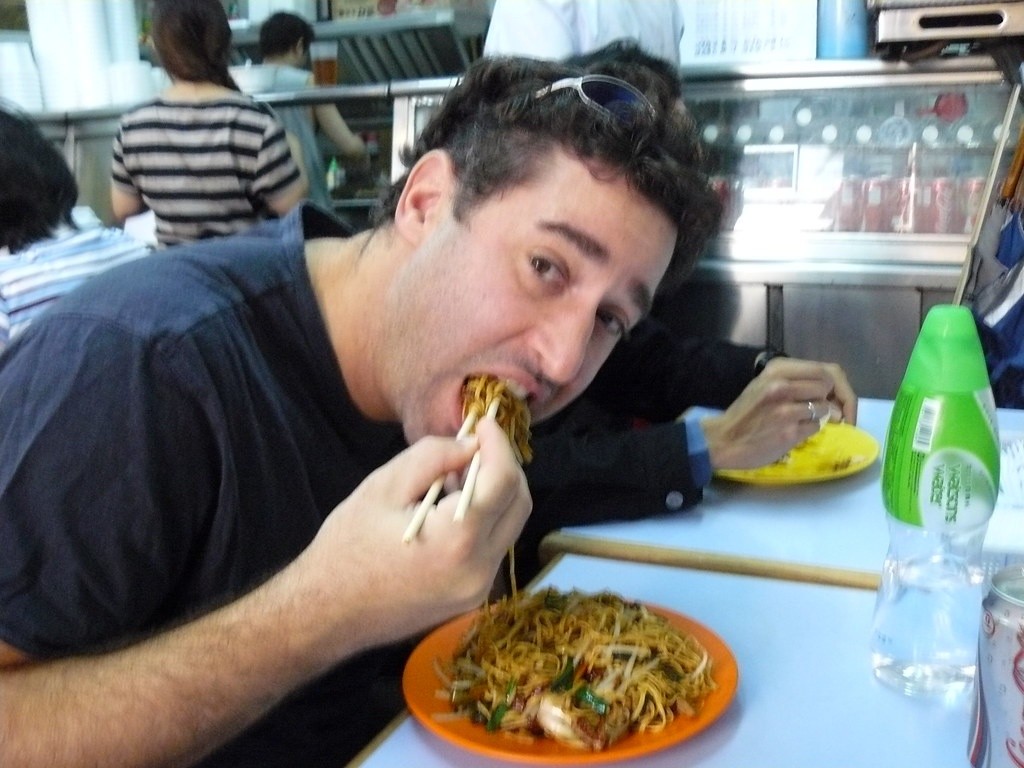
[226,64,279,94]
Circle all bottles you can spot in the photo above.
[228,0,241,20]
[870,303,1002,705]
[701,93,1012,148]
[142,1,155,42]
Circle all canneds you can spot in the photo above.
[834,173,986,237]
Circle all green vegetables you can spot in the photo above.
[484,587,615,730]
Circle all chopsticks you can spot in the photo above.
[539,533,882,592]
[402,392,500,548]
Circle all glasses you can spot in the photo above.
[531,74,657,132]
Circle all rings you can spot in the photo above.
[807,401,816,421]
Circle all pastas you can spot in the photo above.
[456,373,534,587]
[449,585,718,736]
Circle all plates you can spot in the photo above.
[402,592,739,764]
[694,415,878,483]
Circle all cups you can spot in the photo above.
[308,41,338,86]
[817,0,869,59]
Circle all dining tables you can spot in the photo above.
[338,394,1024,768]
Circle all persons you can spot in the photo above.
[0,104,150,343]
[0,59,710,768]
[482,0,685,73]
[531,54,858,529]
[234,11,366,213]
[109,0,310,251]
